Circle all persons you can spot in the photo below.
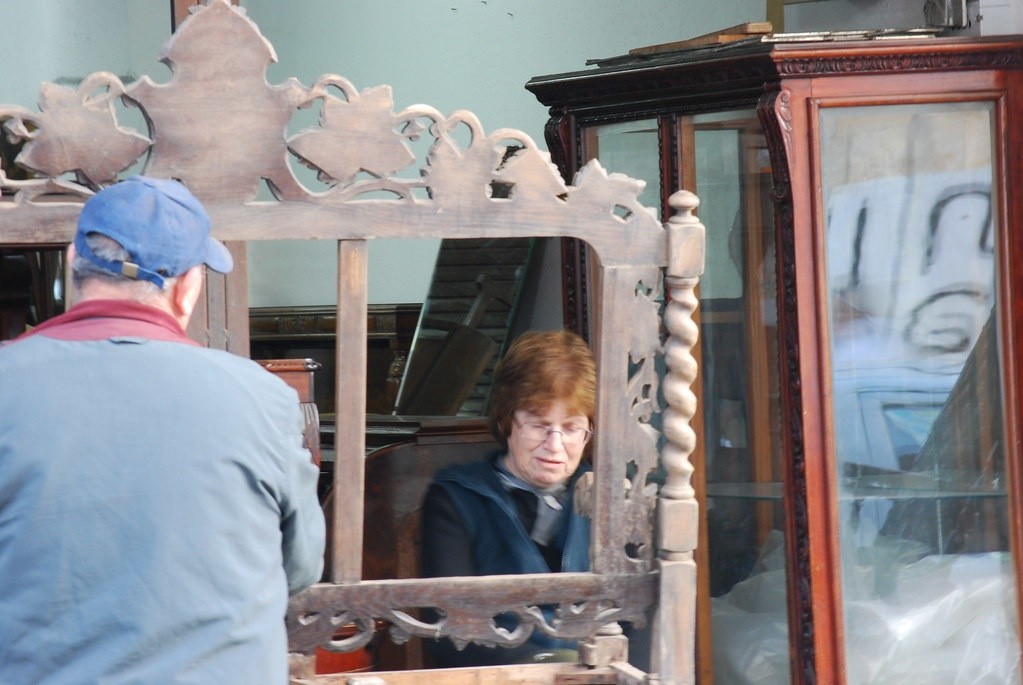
[2,176,327,684]
[418,328,592,670]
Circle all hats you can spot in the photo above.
[74,174,233,290]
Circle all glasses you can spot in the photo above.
[512,415,594,446]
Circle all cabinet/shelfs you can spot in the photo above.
[524,33,1023,685]
[1,0,712,685]
